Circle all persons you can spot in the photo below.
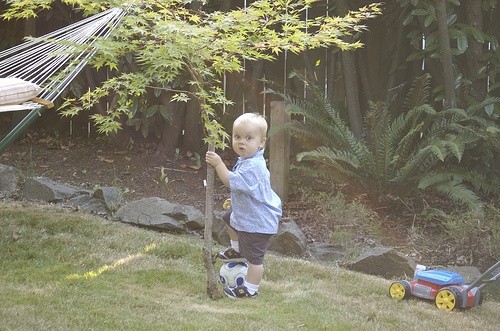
[206,113,282,299]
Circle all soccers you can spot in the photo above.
[219,261,249,288]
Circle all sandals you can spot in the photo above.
[217,247,247,262]
[223,285,258,300]
